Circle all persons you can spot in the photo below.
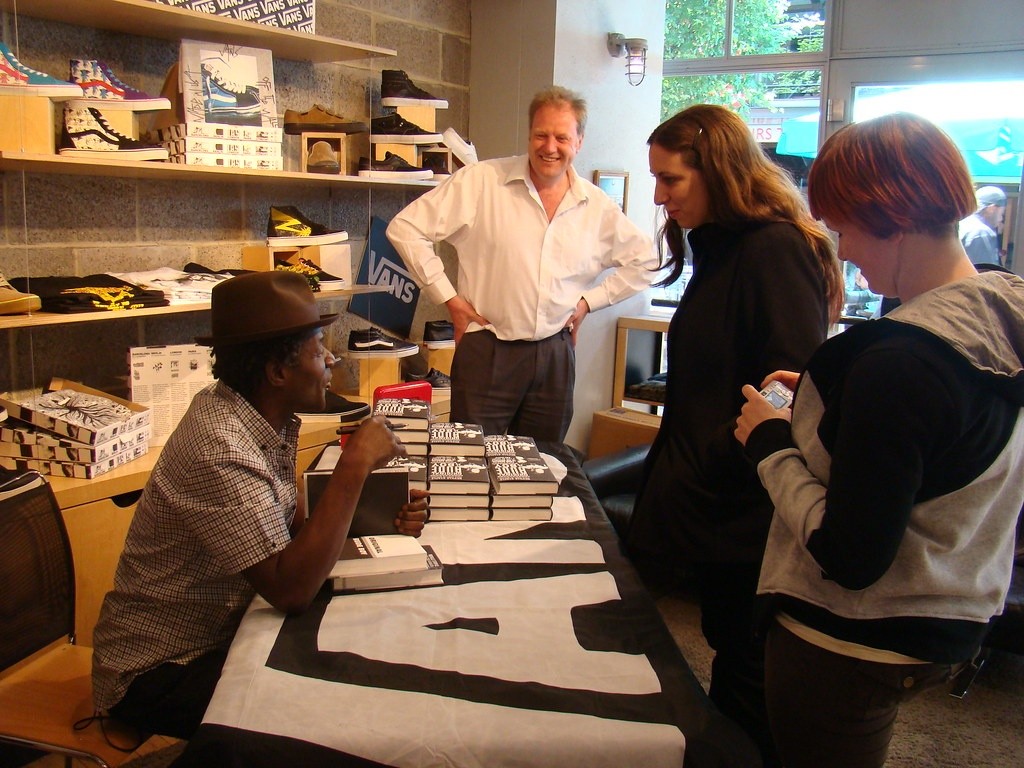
[687,113,1024,768]
[619,105,846,606]
[385,86,665,448]
[92,269,431,735]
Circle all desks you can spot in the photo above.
[196,439,760,767]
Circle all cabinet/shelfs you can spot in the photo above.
[612,310,676,409]
[1,0,451,650]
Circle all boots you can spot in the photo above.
[70,59,171,111]
[382,70,449,109]
[59,107,168,161]
[267,205,348,246]
[0,42,84,96]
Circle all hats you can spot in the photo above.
[194,270,336,346]
[975,186,1007,214]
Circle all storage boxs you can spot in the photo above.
[125,343,218,447]
[587,406,662,459]
[146,122,285,170]
[1,377,151,481]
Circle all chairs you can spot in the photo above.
[0,470,186,768]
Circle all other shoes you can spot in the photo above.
[284,104,368,135]
[1,273,41,313]
[307,141,339,174]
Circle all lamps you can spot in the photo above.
[608,33,649,87]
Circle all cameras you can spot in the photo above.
[758,380,795,410]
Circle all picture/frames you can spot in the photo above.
[593,169,630,216]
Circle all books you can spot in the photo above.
[326,536,444,590]
[372,398,560,521]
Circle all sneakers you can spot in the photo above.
[294,391,371,422]
[278,257,345,291]
[371,111,444,144]
[423,320,456,350]
[358,150,434,180]
[442,127,478,165]
[347,327,419,357]
[405,368,452,395]
